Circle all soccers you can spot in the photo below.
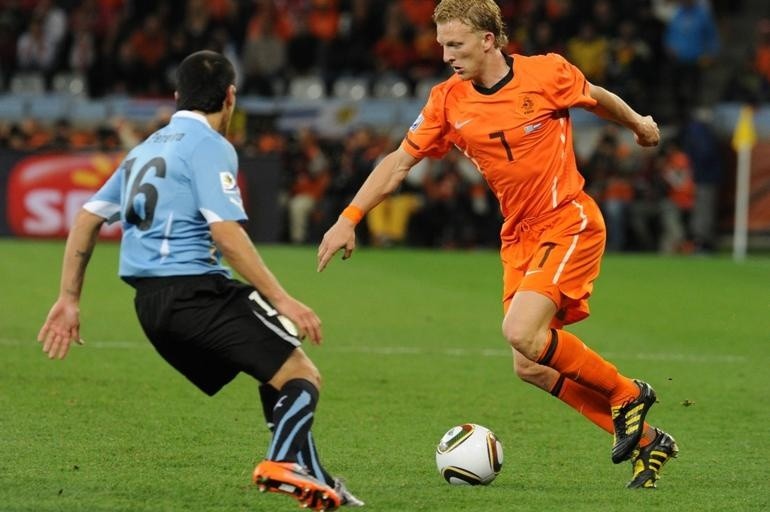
[436,423,503,484]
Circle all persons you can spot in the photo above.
[317,0,678,491]
[1,1,769,255]
[38,49,366,512]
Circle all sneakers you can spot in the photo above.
[609,377,657,464]
[626,427,679,489]
[253,458,365,512]
